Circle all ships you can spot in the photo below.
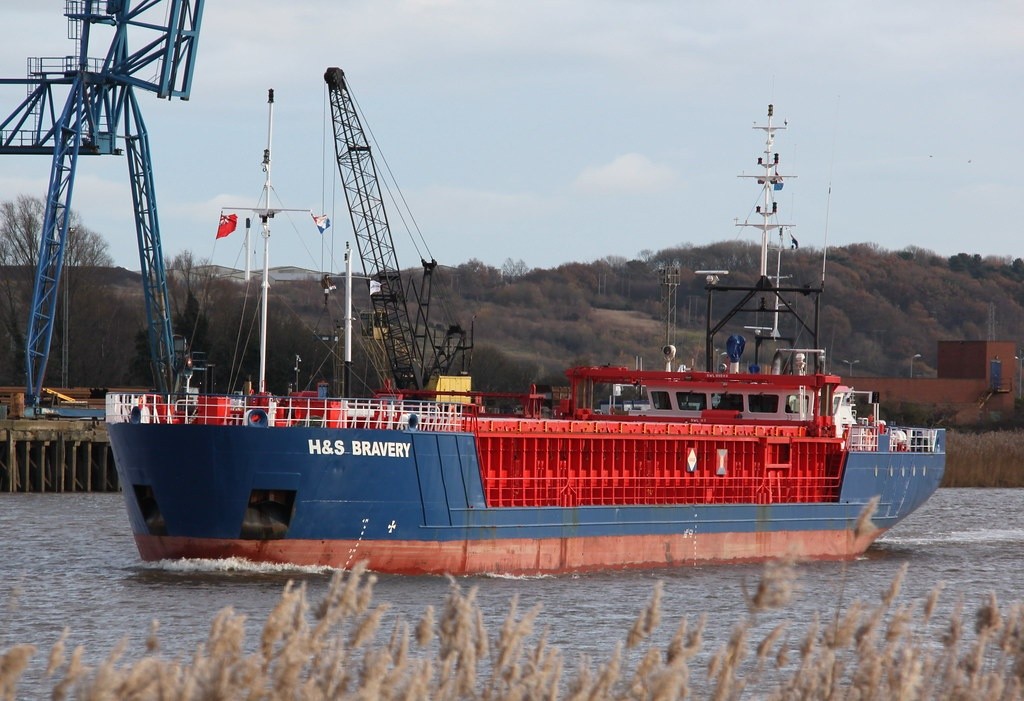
[102,67,949,581]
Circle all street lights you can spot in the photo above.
[910,354,922,377]
[841,358,861,377]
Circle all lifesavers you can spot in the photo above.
[897,442,907,452]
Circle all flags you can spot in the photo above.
[215,214,238,239]
[313,214,331,234]
[791,235,798,250]
[369,280,382,295]
[774,171,784,191]
